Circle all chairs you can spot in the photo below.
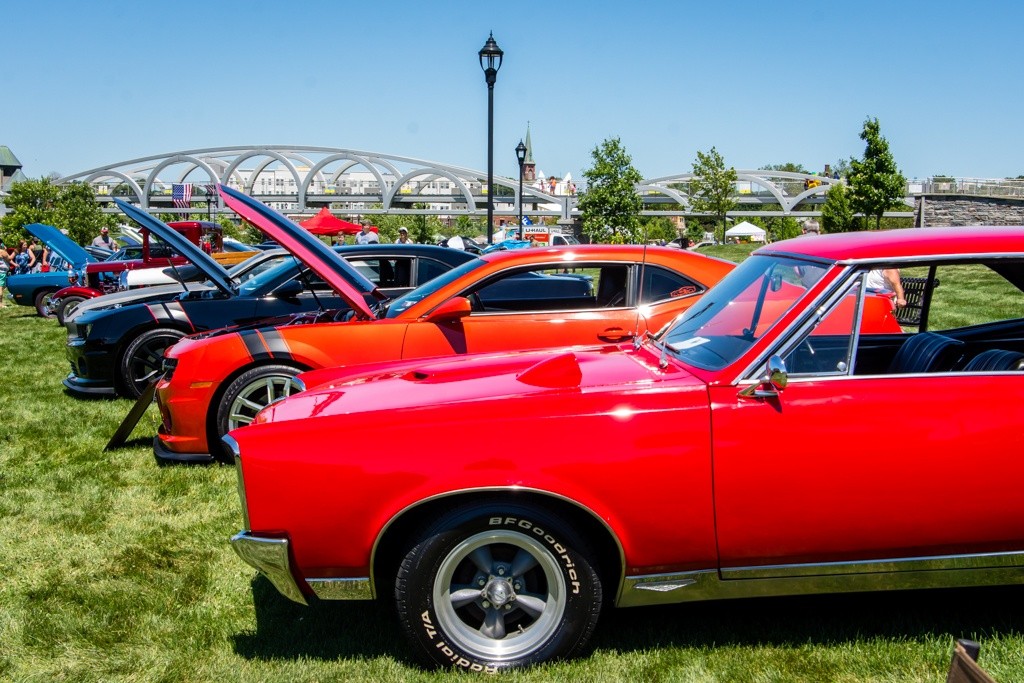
[962,348,1024,372]
[890,333,964,373]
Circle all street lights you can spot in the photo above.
[476,27,504,245]
[514,137,528,240]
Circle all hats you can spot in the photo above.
[398,227,408,234]
[101,226,109,232]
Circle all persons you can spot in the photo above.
[333,231,347,246]
[509,233,522,240]
[530,236,539,248]
[866,267,906,310]
[354,220,379,245]
[793,220,828,287]
[91,227,114,251]
[688,239,695,246]
[395,227,414,244]
[804,172,840,198]
[547,176,576,196]
[0,237,51,308]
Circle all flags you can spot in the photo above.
[204,185,217,205]
[172,183,193,219]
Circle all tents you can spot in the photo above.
[725,220,766,245]
[299,206,378,246]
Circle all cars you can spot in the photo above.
[150,204,906,467]
[221,221,1024,676]
[2,194,601,405]
